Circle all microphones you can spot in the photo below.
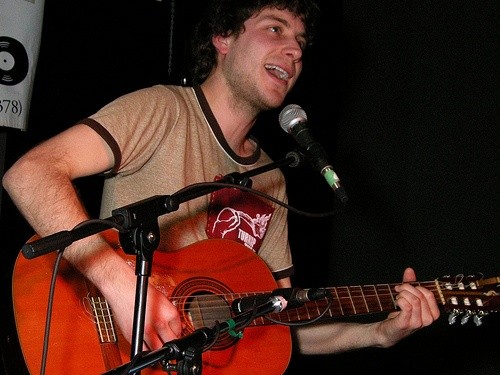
[278,104,348,202]
[231,288,329,317]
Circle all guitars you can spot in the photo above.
[13,226,500,375]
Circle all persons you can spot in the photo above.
[2,0,439,375]
